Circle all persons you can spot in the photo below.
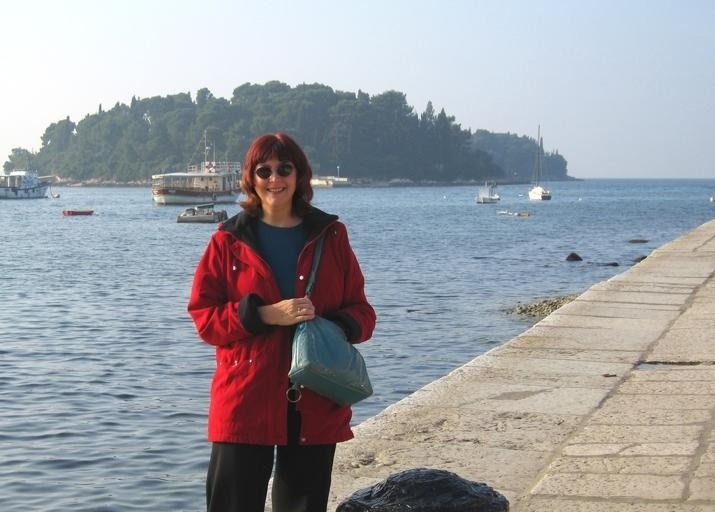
[186,132,378,511]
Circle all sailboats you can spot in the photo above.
[527,123,550,200]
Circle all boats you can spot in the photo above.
[496,209,530,217]
[63,210,94,217]
[0,169,52,199]
[176,203,227,224]
[151,130,244,206]
[474,181,500,203]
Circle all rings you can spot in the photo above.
[296,307,301,316]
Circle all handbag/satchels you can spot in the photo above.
[287,314,373,408]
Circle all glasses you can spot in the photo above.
[253,164,294,180]
